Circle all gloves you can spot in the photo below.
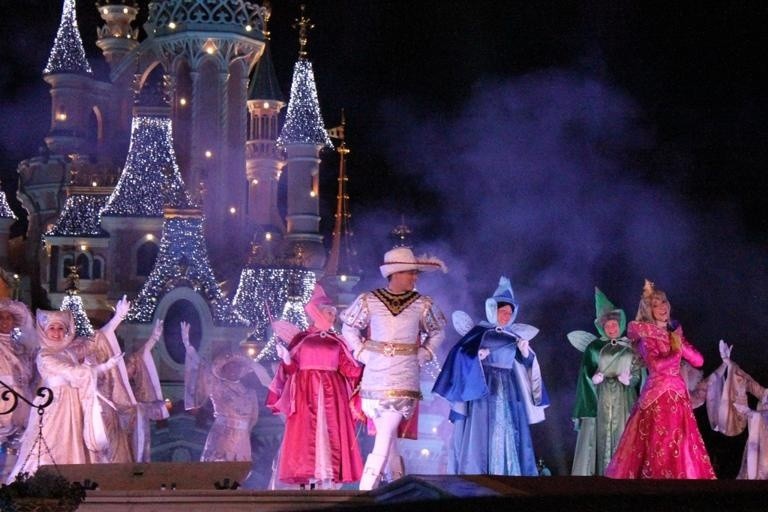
[116,294,132,320]
[718,340,727,359]
[725,343,733,358]
[618,368,632,385]
[592,372,604,385]
[478,349,491,360]
[180,320,191,347]
[151,318,164,340]
[276,343,291,365]
[669,332,681,350]
[515,337,530,358]
[101,352,126,371]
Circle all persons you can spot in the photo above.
[338,246,446,491]
[677,357,726,411]
[606,280,718,480]
[718,339,768,478]
[567,287,640,477]
[432,276,551,475]
[0,279,174,485]
[179,319,260,480]
[265,284,365,489]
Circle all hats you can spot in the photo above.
[593,286,626,338]
[304,282,332,330]
[487,275,517,305]
[379,247,448,277]
